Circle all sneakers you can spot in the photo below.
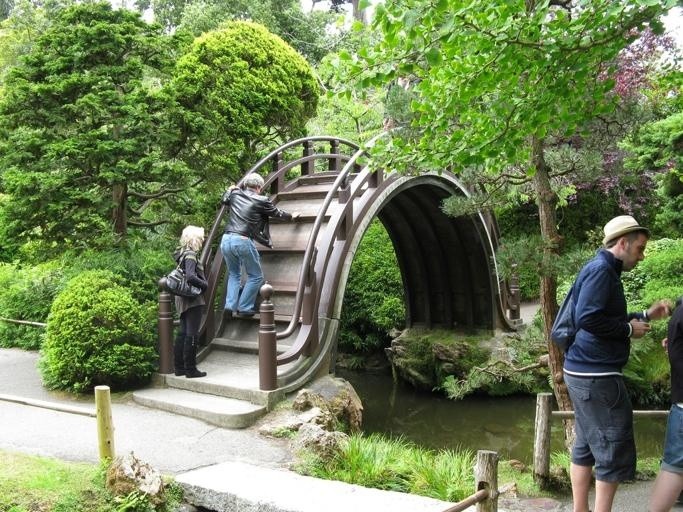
[238,310,259,315]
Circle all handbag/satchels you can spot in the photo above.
[551,286,580,351]
[165,270,202,299]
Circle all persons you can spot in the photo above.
[549,215,673,511]
[171,225,206,376]
[215,171,300,319]
[647,293,681,511]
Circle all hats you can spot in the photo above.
[602,215,651,247]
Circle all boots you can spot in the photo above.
[174,333,207,377]
